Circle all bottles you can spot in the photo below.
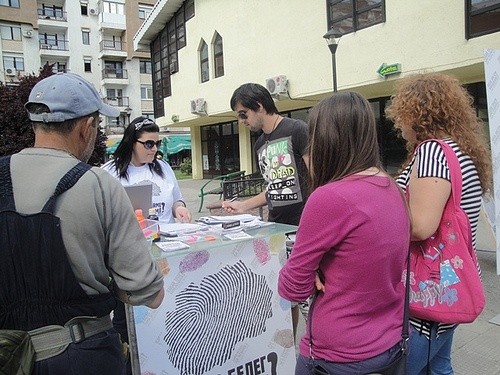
[135,209,144,222]
[147,208,161,242]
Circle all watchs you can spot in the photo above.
[221,83,314,345]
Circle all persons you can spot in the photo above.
[1,72,164,375]
[100,117,192,222]
[383,72,492,375]
[279,90,411,375]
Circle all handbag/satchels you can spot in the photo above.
[307,346,409,375]
[405,139,487,324]
[0,328,36,375]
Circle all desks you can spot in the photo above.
[124,222,298,375]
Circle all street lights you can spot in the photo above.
[322,25,342,93]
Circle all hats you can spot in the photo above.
[23,72,120,123]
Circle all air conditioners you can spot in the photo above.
[265,75,288,96]
[4,67,16,76]
[190,98,206,113]
[23,30,33,37]
[88,8,99,15]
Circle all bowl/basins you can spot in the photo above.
[139,219,159,239]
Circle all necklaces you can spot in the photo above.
[266,116,279,144]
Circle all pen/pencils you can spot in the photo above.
[220,197,237,210]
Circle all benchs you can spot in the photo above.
[198,171,245,212]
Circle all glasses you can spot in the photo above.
[136,139,162,149]
[237,112,248,119]
